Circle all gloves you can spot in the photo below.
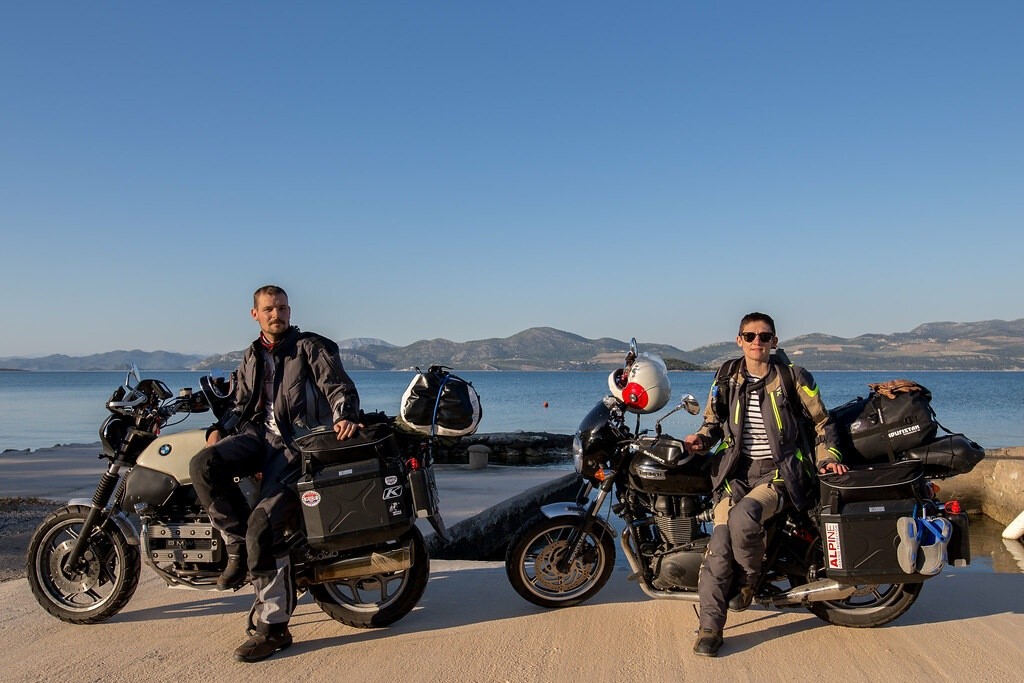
[868,380,921,399]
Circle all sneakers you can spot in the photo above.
[725,568,763,612]
[693,626,723,655]
[216,543,247,590]
[233,619,292,663]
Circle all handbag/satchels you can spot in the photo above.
[295,423,395,474]
[829,383,937,461]
[947,510,971,567]
[400,365,483,437]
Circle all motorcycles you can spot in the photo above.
[26,361,454,627]
[505,338,970,628]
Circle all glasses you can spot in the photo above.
[739,331,774,343]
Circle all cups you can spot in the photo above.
[179,387,192,396]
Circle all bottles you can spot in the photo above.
[944,501,971,566]
[399,439,434,518]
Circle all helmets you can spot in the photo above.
[608,354,671,414]
[199,372,236,418]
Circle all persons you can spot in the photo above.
[683,313,849,657]
[189,285,364,661]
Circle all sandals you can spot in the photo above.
[897,517,923,574]
[916,517,952,575]
